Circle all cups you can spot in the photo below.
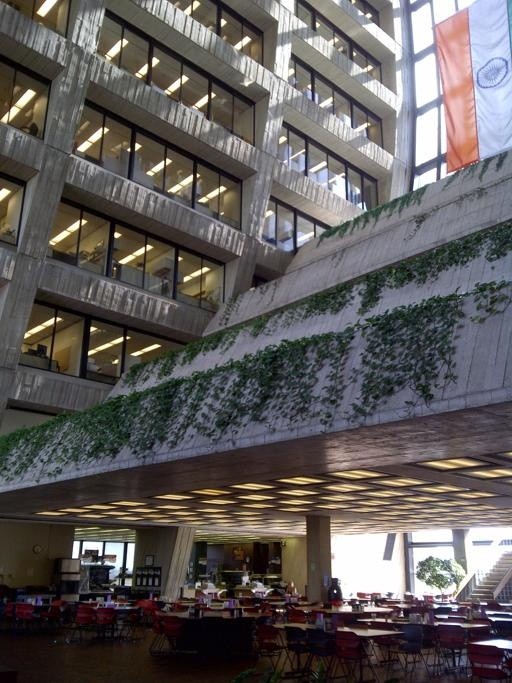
[285,592,299,603]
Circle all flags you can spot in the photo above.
[430,0,512,174]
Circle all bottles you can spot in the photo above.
[189,592,246,618]
[88,594,129,609]
[149,592,174,603]
[371,608,436,626]
[24,594,42,606]
[351,603,364,613]
[465,605,489,620]
[315,612,339,632]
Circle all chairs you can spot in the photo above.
[1,586,162,644]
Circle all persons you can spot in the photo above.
[239,555,266,579]
[267,580,285,595]
[283,579,298,596]
